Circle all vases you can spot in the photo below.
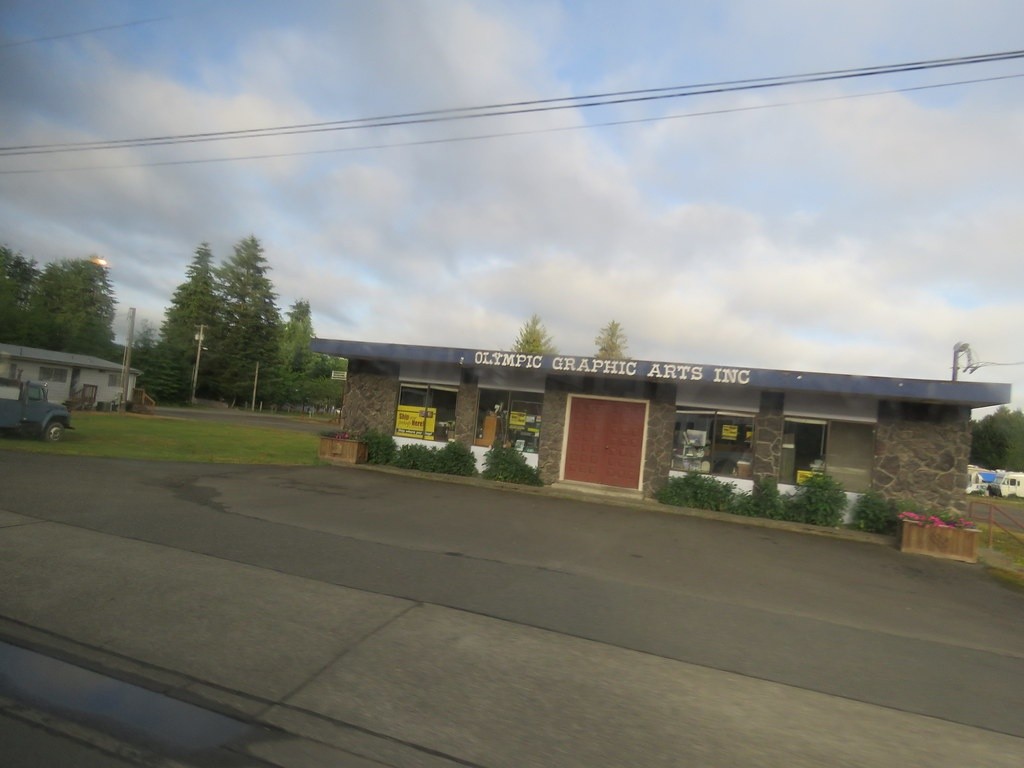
[896,519,982,563]
[318,437,368,464]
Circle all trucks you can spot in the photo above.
[967,463,1024,496]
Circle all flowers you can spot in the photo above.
[897,498,975,529]
[315,428,366,440]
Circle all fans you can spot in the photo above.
[494,402,505,415]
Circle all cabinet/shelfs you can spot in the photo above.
[670,443,711,474]
[475,417,501,446]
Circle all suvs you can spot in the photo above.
[0,377,76,445]
